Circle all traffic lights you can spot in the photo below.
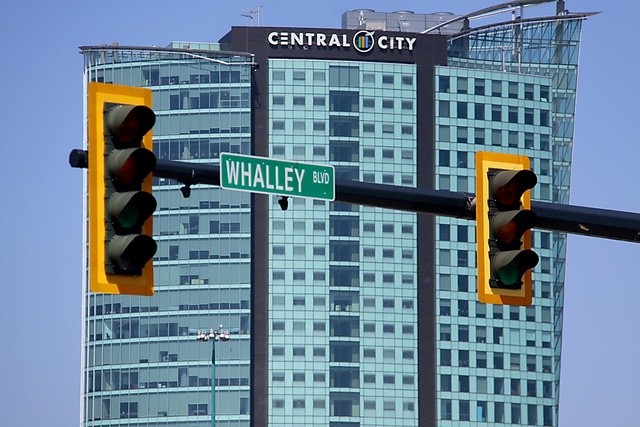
[487,167,538,289]
[102,101,157,277]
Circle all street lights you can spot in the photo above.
[196,328,231,426]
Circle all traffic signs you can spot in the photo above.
[220,152,335,203]
